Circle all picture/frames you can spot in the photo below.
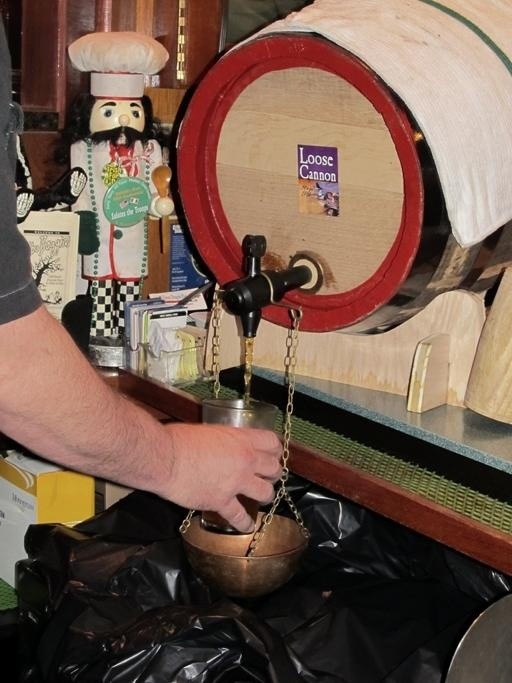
[13,212,81,325]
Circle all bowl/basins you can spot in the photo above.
[182,511,311,597]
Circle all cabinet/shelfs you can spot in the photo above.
[16,0,227,132]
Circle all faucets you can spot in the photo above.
[221,265,314,340]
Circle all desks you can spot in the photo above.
[111,366,512,580]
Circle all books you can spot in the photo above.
[122,295,189,376]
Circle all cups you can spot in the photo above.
[200,397,280,536]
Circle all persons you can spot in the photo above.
[1,25,286,532]
[46,29,175,374]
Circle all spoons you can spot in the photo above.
[151,165,173,253]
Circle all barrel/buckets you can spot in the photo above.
[169,31,512,335]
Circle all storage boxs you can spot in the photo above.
[0,447,95,591]
[91,477,134,515]
